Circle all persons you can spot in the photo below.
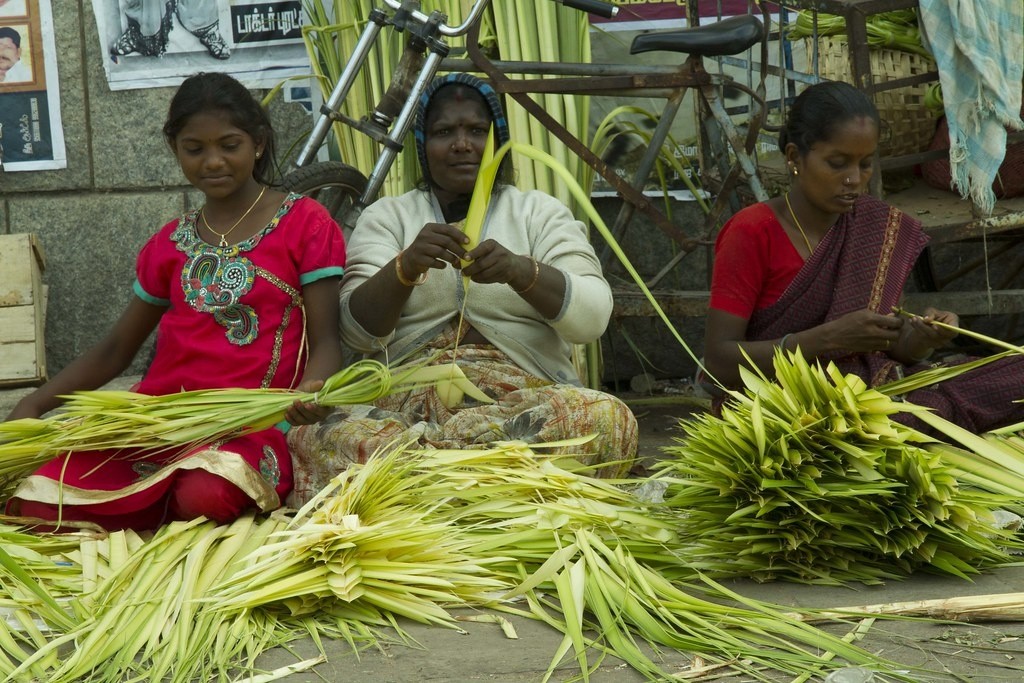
[0,27,31,83]
[288,74,639,508]
[697,82,1024,448]
[112,0,231,59]
[0,73,347,531]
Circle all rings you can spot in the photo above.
[886,340,890,347]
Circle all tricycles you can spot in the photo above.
[267,0,1022,359]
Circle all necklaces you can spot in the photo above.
[202,186,266,247]
[785,192,813,253]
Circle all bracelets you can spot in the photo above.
[508,255,538,294]
[780,334,793,349]
[396,250,429,286]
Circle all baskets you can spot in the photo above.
[806,38,942,173]
